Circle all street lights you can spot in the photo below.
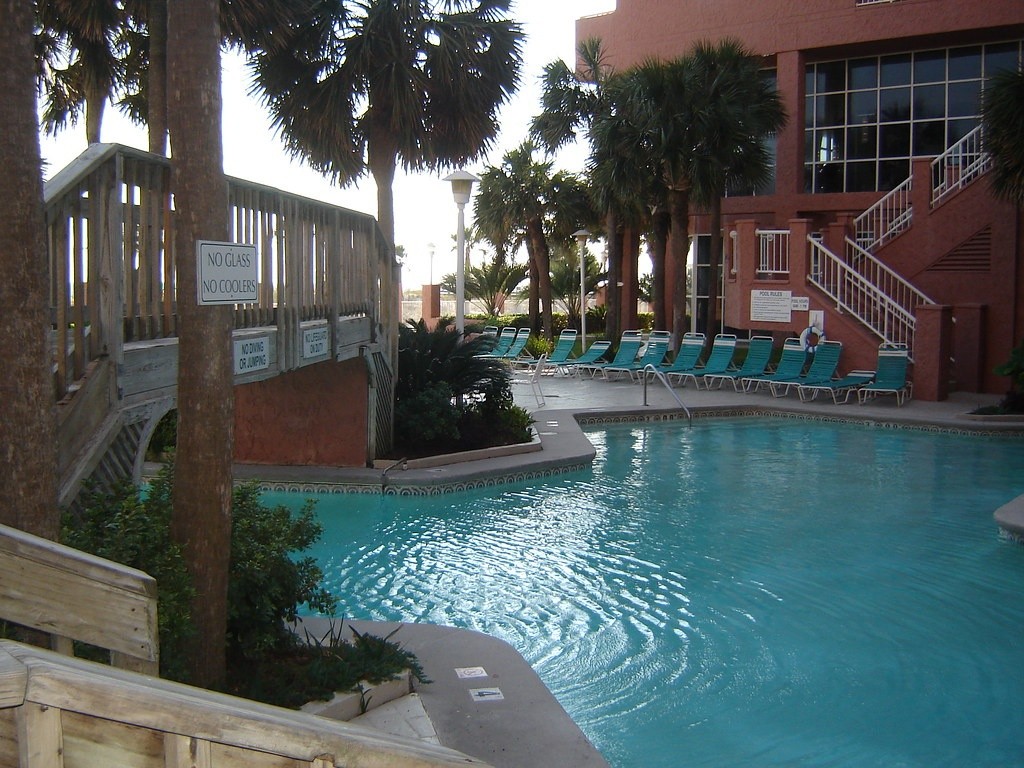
[443,170,483,345]
[426,243,436,285]
[572,229,591,354]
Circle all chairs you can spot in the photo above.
[472,326,913,408]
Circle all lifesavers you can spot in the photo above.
[799,326,826,353]
